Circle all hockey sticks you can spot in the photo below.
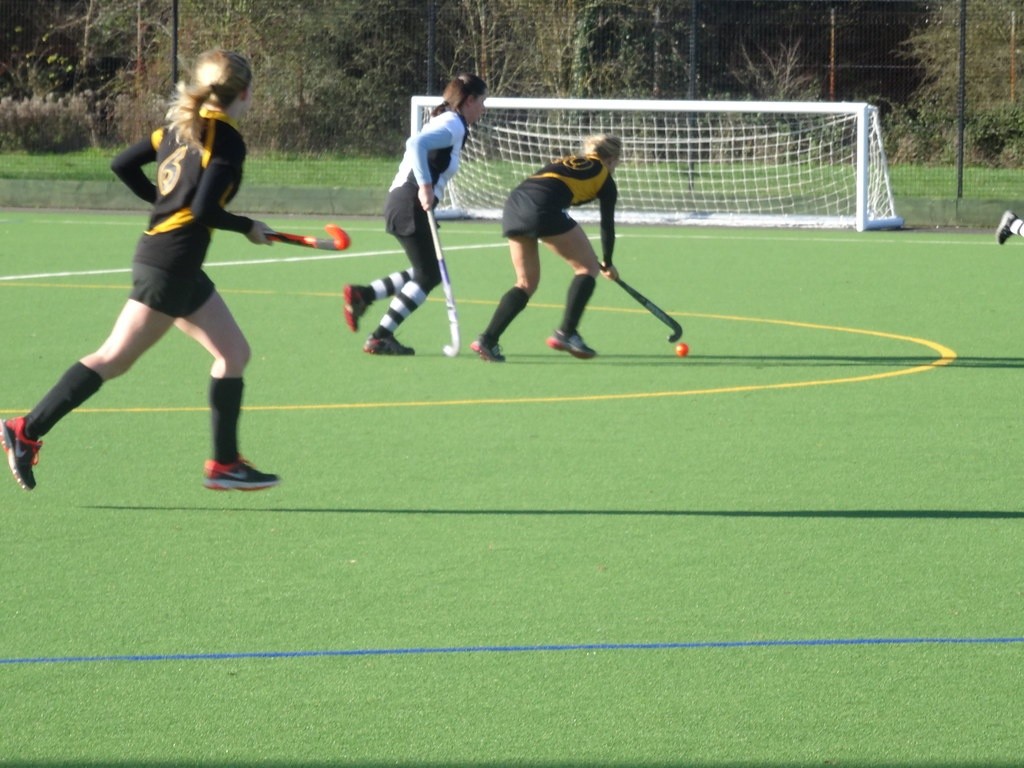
[598,261,683,344]
[427,206,460,357]
[264,224,350,251]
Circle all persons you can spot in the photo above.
[0,49,279,492]
[471,134,624,362]
[996,210,1024,244]
[345,73,489,356]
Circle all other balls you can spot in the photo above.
[675,342,689,357]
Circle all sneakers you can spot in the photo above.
[996,211,1017,245]
[204,457,282,491]
[0,416,43,491]
[471,334,506,362]
[363,334,414,356]
[343,284,369,332]
[546,329,596,359]
